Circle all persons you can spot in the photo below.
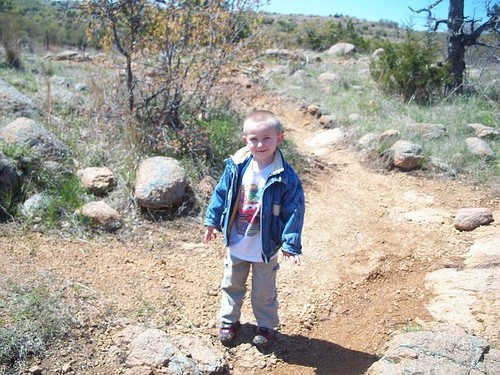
[203,109,305,351]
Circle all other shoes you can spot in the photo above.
[219,321,240,345]
[252,326,276,348]
